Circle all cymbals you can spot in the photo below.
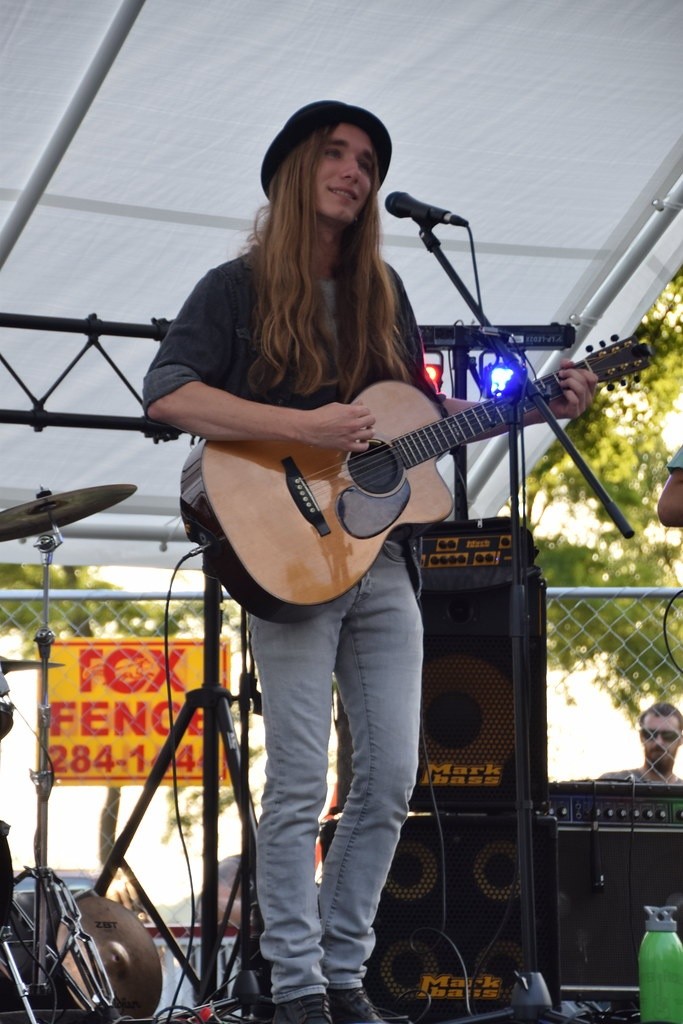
[1,654,66,677]
[0,483,138,541]
[55,895,162,1022]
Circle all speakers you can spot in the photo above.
[317,518,558,1015]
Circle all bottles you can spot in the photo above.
[638,903,682,1024]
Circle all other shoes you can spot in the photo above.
[273,988,392,1024]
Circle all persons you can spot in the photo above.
[595,703,682,783]
[145,100,597,1023]
[657,443,683,526]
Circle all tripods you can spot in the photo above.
[92,547,267,996]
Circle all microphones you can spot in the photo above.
[384,191,470,229]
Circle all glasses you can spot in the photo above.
[639,728,681,743]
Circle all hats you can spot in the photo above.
[260,100,393,199]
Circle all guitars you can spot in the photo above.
[179,333,654,626]
